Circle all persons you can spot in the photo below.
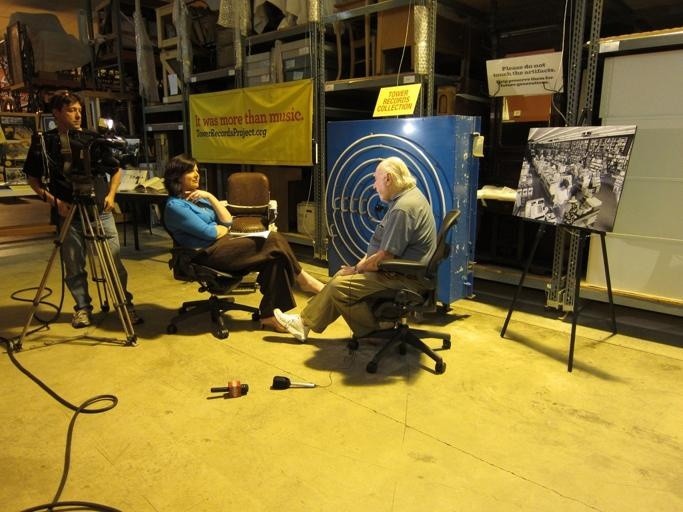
[552,178,570,209]
[570,164,578,180]
[21,91,145,329]
[162,151,326,334]
[273,155,438,344]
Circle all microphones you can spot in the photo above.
[273,373,316,389]
[209,380,250,400]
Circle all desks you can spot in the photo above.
[113,190,171,251]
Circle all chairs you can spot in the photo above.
[341,211,462,376]
[159,173,278,326]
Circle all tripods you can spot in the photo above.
[12,175,143,354]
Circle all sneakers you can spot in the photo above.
[71,307,92,328]
[121,304,143,326]
[273,307,310,343]
